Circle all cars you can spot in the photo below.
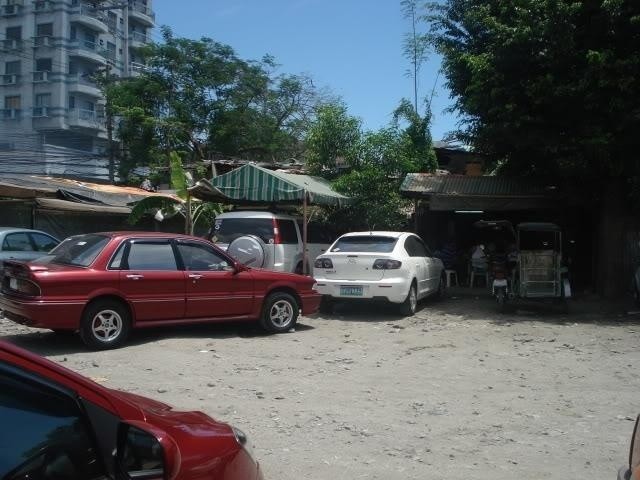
[0,227,65,256]
[310,230,450,316]
[0,230,321,352]
[0,337,266,480]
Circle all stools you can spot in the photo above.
[443,269,458,288]
[469,272,489,288]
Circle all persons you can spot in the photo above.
[439,238,516,288]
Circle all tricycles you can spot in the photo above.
[489,222,574,313]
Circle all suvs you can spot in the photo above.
[213,209,333,276]
